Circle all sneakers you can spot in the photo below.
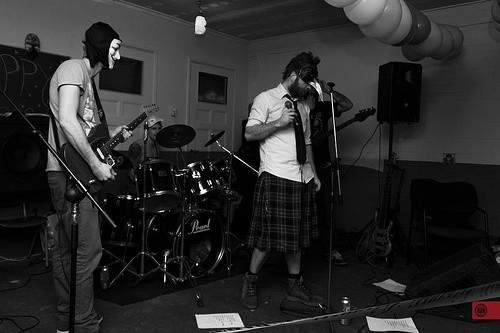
[285,277,325,306]
[240,272,259,311]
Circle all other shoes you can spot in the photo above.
[322,249,346,265]
[56,312,103,333]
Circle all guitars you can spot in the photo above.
[313,106,376,145]
[59,103,159,192]
[367,174,393,258]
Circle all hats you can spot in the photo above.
[145,116,163,129]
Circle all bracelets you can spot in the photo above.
[329,88,332,93]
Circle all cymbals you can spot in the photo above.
[205,130,225,147]
[158,124,196,148]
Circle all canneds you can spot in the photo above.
[99,265,110,288]
[340,297,352,326]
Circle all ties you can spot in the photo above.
[284,94,307,166]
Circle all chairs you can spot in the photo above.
[0,191,48,269]
[409,178,488,276]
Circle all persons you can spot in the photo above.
[239,52,325,312]
[298,78,353,265]
[128,116,164,151]
[45,21,134,333]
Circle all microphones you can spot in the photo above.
[285,101,299,126]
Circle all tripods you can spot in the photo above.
[102,122,260,308]
[287,91,344,333]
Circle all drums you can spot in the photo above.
[145,205,227,277]
[185,161,226,202]
[135,158,182,213]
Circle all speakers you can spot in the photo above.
[377,61,423,124]
[405,243,500,322]
[0,112,51,209]
